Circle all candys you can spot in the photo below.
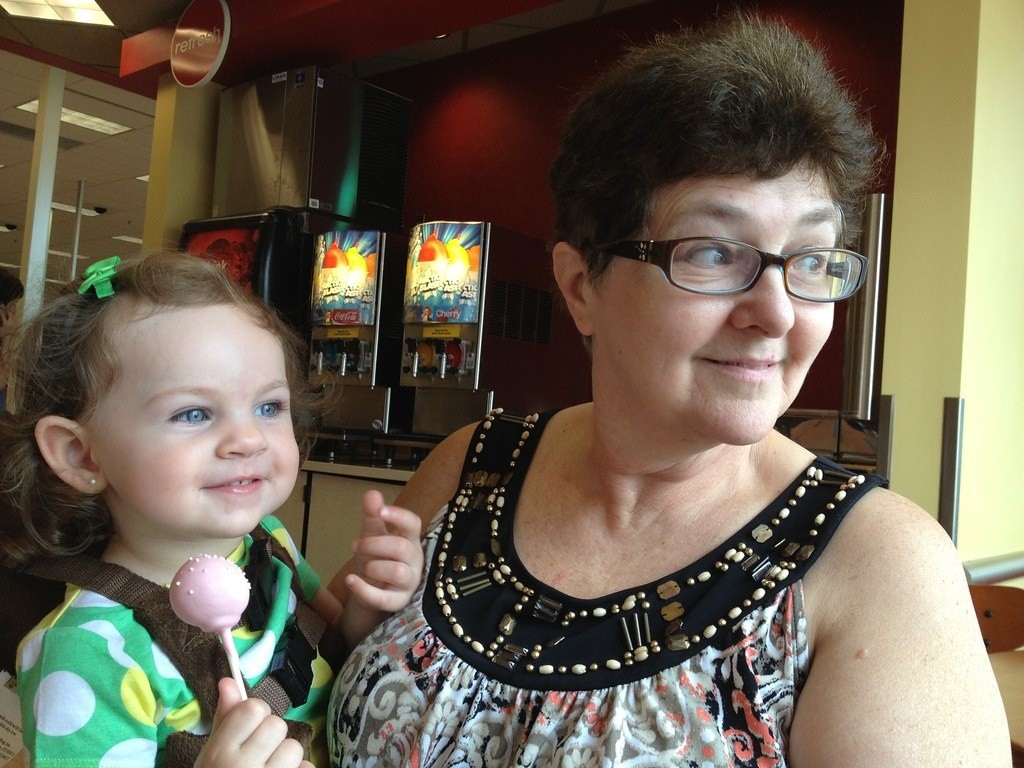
[170,553,254,702]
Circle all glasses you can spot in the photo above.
[598,235,867,302]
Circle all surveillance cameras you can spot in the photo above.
[95,206,107,214]
[6,224,16,230]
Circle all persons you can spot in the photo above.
[16,255,424,768]
[326,46,1015,768]
